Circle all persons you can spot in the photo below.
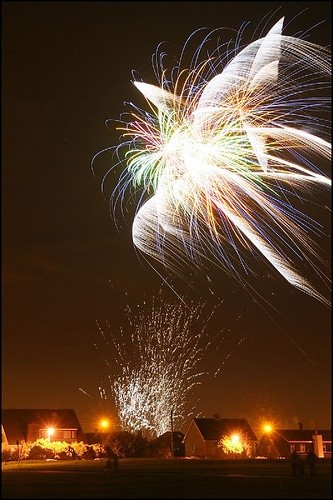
[290,450,300,480]
[297,457,306,476]
[305,448,318,476]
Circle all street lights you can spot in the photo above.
[231,434,240,459]
[262,423,274,458]
[100,418,109,447]
[47,426,54,444]
